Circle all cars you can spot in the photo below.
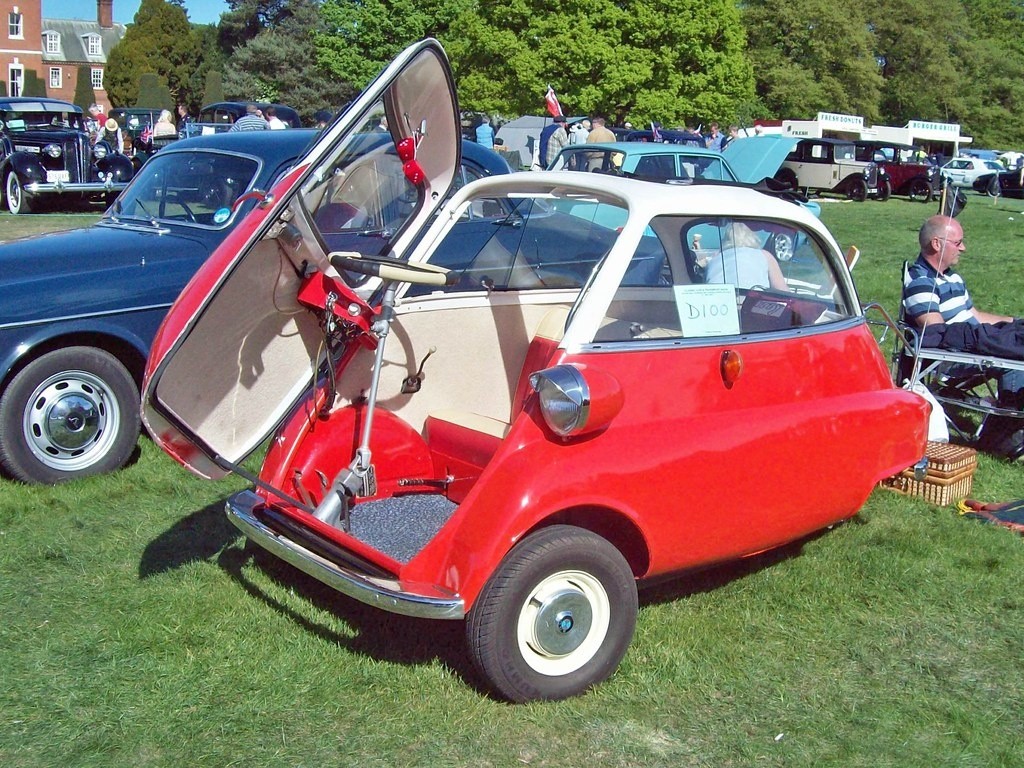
[533,134,832,265]
[0,98,135,218]
[940,157,1009,190]
[131,35,936,709]
[959,148,998,161]
[779,136,893,203]
[850,140,946,205]
[972,165,1024,198]
[625,130,720,183]
[1,131,551,489]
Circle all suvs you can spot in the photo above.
[108,103,303,209]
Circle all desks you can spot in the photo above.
[906,344,1023,421]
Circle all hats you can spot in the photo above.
[105,118,118,131]
[554,116,567,122]
[569,125,578,132]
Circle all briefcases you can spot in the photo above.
[879,441,976,506]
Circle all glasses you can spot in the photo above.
[939,237,962,247]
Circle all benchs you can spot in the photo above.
[427,304,684,441]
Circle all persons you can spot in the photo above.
[915,146,928,165]
[95,118,124,155]
[936,150,946,167]
[475,116,495,150]
[154,110,177,135]
[376,116,389,132]
[177,105,193,132]
[618,120,766,154]
[1020,162,1024,214]
[703,222,793,293]
[227,104,287,132]
[903,215,1024,411]
[539,115,617,173]
[87,102,108,131]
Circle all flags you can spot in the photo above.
[545,86,567,127]
[140,125,152,144]
[651,123,662,142]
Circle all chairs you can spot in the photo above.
[890,261,998,442]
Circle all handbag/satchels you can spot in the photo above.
[903,379,950,444]
